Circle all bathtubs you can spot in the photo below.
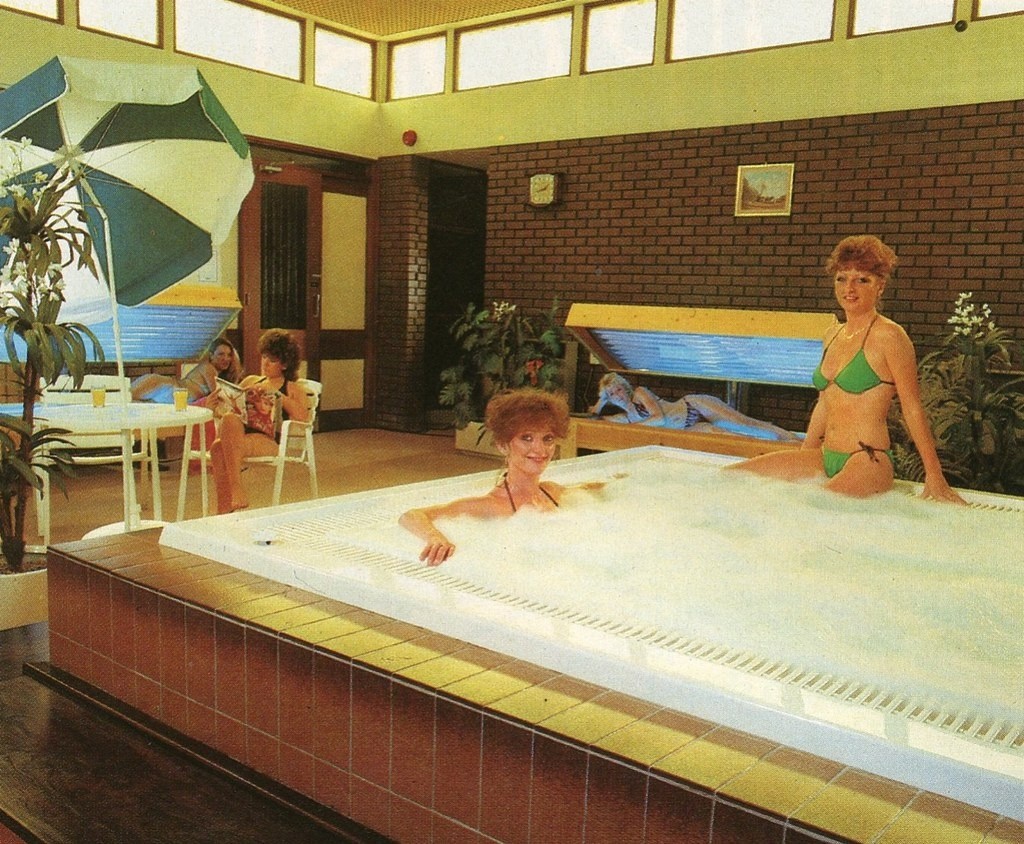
[42,445,1024,844]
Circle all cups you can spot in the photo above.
[173,388,188,411]
[91,384,106,408]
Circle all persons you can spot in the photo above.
[721,234,968,505]
[586,371,804,443]
[396,386,575,567]
[128,336,236,404]
[202,329,307,515]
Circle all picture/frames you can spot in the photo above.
[734,163,796,217]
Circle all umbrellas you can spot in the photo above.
[0,51,257,530]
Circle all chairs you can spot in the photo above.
[33,375,323,546]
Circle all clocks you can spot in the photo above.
[528,173,563,208]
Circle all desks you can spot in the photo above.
[39,403,213,537]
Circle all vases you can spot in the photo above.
[0,544,49,631]
[455,422,560,461]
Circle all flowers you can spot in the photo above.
[440,295,567,447]
[887,292,1024,497]
[0,134,104,571]
